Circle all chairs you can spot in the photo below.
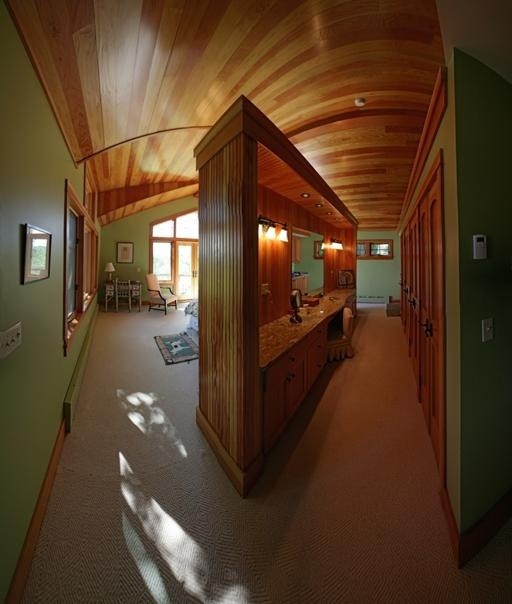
[145,273,177,315]
[115,280,131,312]
[327,306,355,362]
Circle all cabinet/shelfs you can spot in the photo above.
[261,321,328,456]
[398,147,450,492]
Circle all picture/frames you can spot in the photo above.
[116,242,134,264]
[21,222,52,286]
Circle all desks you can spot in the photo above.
[103,280,142,312]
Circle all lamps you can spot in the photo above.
[257,214,289,244]
[330,239,343,250]
[104,262,116,282]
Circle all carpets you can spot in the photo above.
[154,330,200,364]
